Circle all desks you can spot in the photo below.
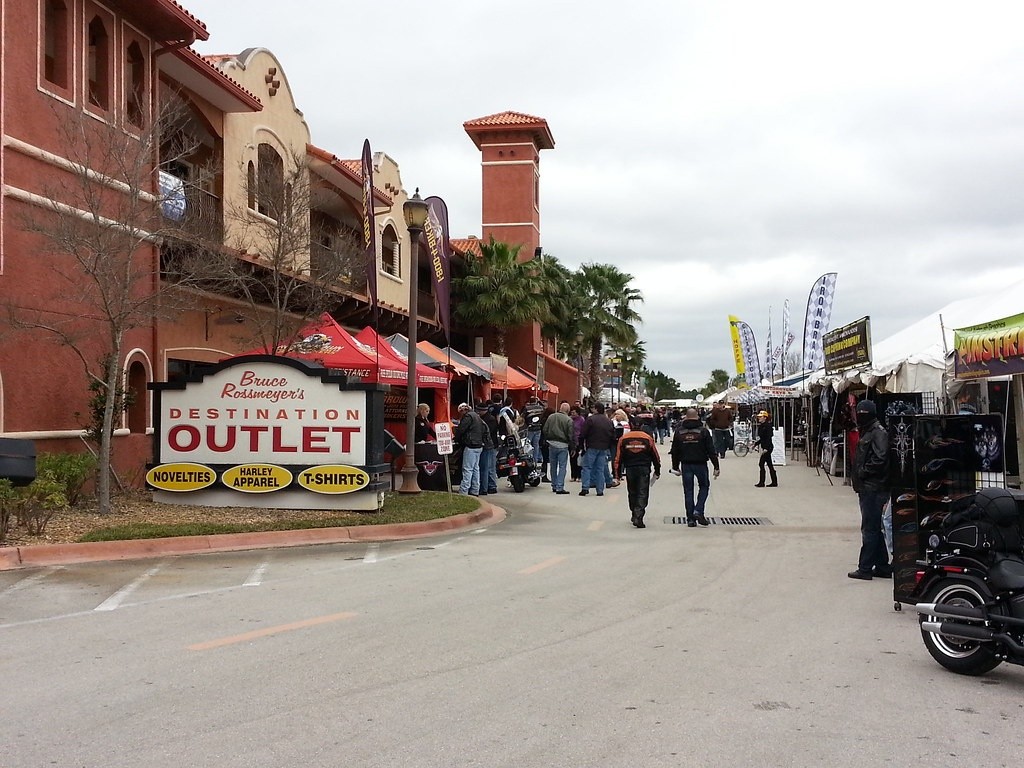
[412,441,464,491]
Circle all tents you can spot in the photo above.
[354,325,560,414]
[218,311,453,418]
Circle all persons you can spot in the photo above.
[847,400,915,580]
[612,417,661,527]
[451,400,735,497]
[668,409,720,526]
[414,403,437,444]
[752,410,778,488]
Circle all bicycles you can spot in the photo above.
[732,434,759,458]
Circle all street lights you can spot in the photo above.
[394,186,423,495]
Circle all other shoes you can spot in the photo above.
[570,478,581,482]
[579,489,589,496]
[755,484,765,487]
[606,481,620,488]
[766,484,777,487]
[479,488,497,495]
[871,569,892,578]
[597,494,603,496]
[631,517,645,528]
[553,489,570,494]
[848,569,872,580]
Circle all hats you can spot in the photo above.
[857,400,876,413]
[475,403,488,409]
[719,400,724,403]
[631,416,644,428]
[757,411,768,417]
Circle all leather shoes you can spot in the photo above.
[693,510,708,526]
[689,521,697,527]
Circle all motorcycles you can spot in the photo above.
[500,416,546,494]
[906,548,1024,677]
